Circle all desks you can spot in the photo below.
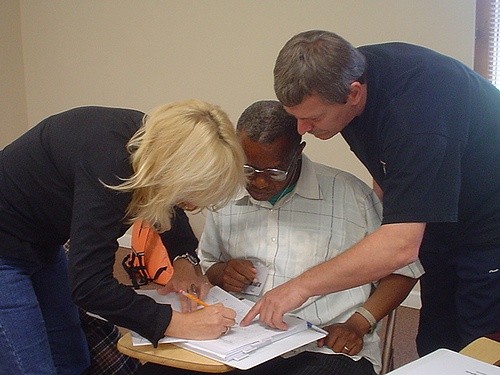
[117,331,233,374]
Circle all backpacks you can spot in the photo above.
[121,216,174,287]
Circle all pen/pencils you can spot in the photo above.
[295,317,349,350]
[179,290,209,308]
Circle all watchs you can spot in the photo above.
[173,251,201,267]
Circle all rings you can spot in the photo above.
[344,346,351,351]
[188,284,197,295]
[224,326,231,335]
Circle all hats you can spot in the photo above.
[238,150,299,181]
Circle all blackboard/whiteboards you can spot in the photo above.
[18,0,477,309]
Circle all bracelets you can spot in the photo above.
[355,307,377,335]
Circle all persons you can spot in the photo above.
[240,30,500,358]
[0,106,250,375]
[143,100,426,375]
